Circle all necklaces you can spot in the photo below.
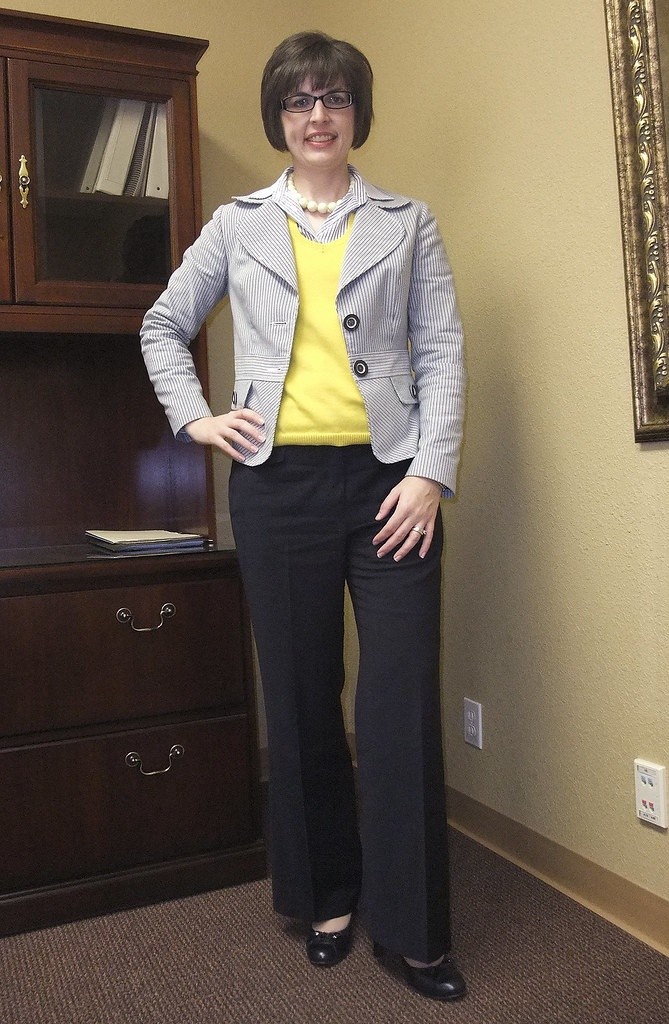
[285,165,357,216]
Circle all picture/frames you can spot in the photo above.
[605,0,669,446]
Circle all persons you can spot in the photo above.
[140,31,465,1007]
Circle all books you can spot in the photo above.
[83,527,215,560]
[79,97,170,200]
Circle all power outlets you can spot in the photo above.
[633,759,668,830]
[463,697,483,751]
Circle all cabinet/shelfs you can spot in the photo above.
[0,7,280,937]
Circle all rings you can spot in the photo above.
[411,523,429,537]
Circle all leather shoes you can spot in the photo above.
[306,906,357,966]
[373,942,468,1000]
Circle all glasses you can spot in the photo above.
[280,90,354,113]
